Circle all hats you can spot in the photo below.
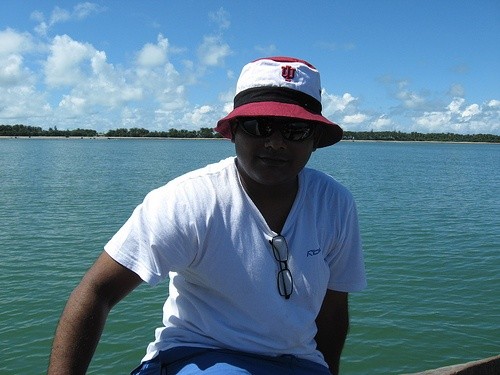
[215,56,343,149]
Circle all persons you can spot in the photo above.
[47,54,367,375]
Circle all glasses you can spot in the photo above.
[234,118,319,143]
[269,233,295,301]
